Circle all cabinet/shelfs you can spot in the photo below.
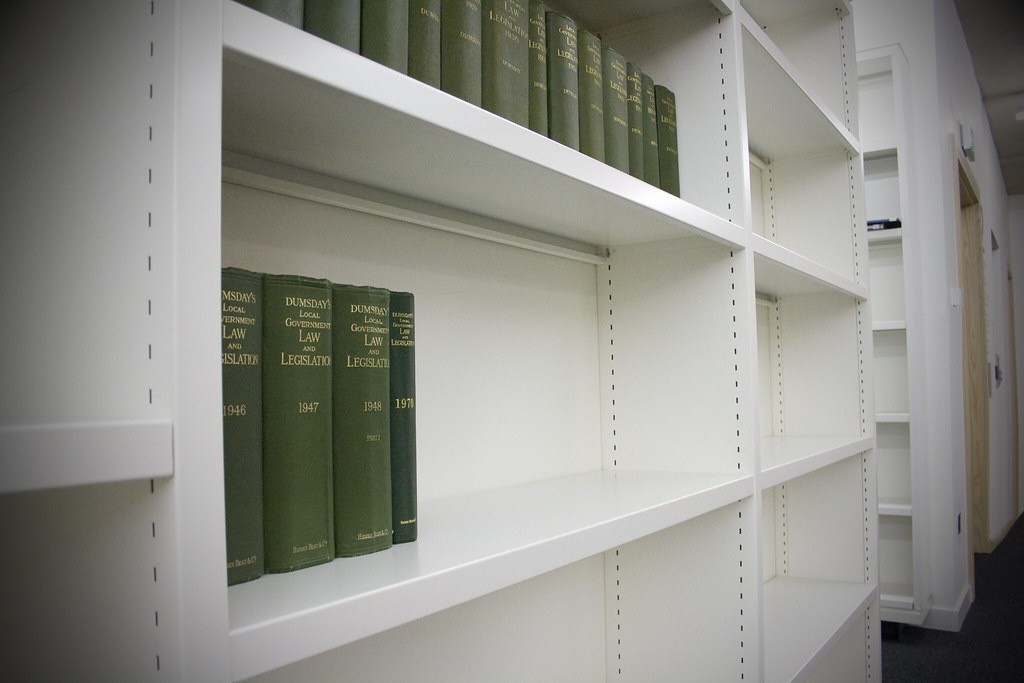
[856,42,928,628]
[0,0,882,682]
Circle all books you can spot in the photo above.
[868,222,903,230]
[867,217,901,225]
[383,287,418,547]
[220,265,267,588]
[255,270,336,576]
[235,0,682,200]
[324,280,393,558]
